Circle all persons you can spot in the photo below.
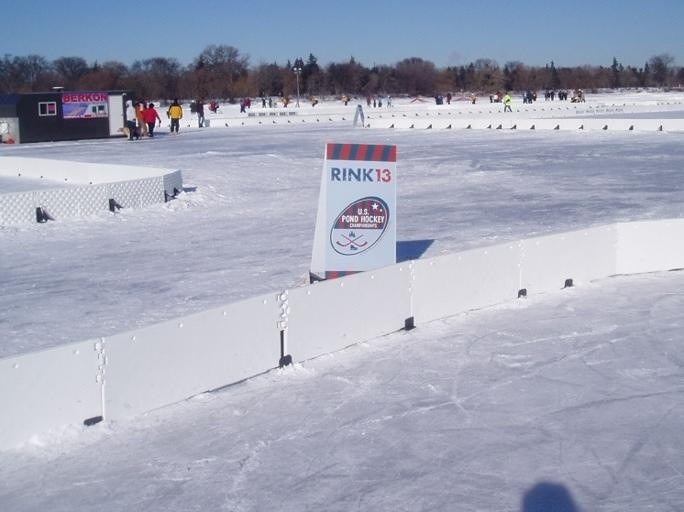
[344,96,347,106]
[488,87,586,112]
[196,99,204,128]
[471,93,476,104]
[166,98,183,135]
[239,94,289,113]
[135,100,147,140]
[210,95,216,113]
[446,93,452,104]
[367,93,392,108]
[144,103,161,138]
[312,96,315,107]
[125,99,139,141]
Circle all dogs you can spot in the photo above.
[116,125,146,140]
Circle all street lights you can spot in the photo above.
[293,66,303,108]
